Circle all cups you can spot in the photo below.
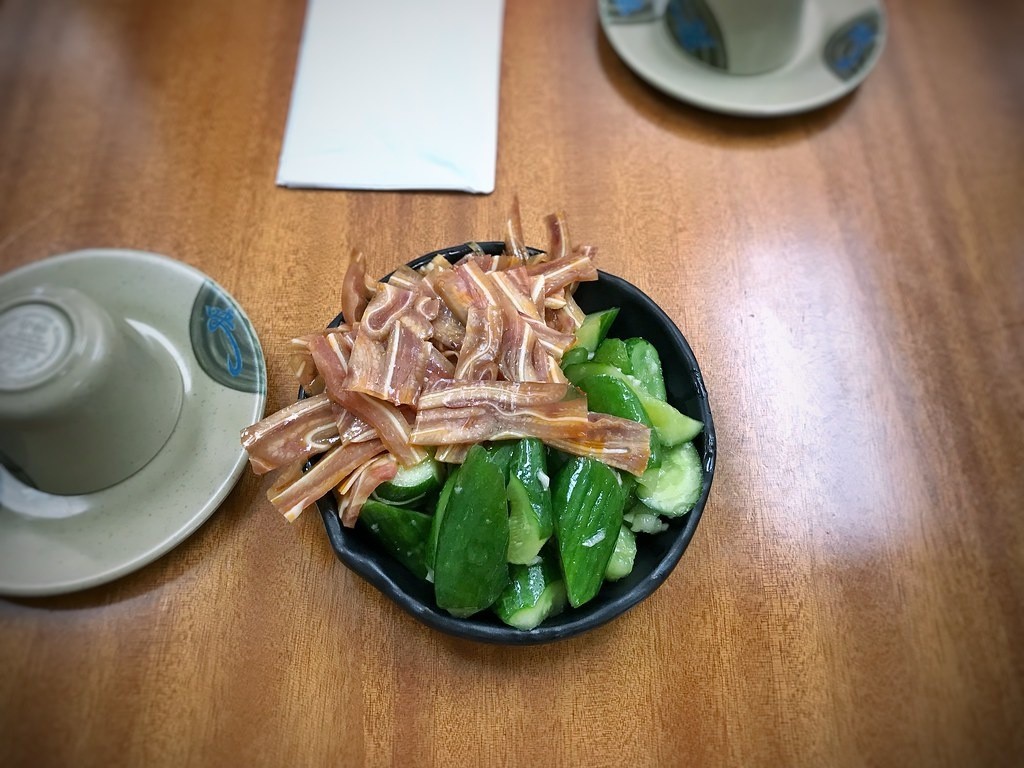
[667,0,808,74]
[0,288,184,495]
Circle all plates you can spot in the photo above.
[597,0,888,115]
[0,249,268,600]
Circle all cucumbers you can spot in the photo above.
[353,307,704,630]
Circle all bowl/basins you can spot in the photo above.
[297,242,718,645]
